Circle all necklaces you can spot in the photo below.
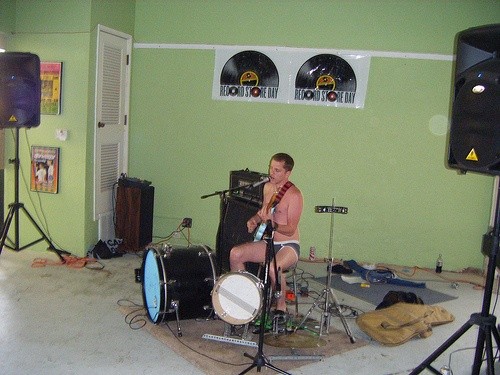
[273,185,278,193]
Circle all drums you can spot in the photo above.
[210,270,264,327]
[141,244,221,324]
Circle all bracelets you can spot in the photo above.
[272,222,278,231]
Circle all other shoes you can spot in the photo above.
[252,325,270,334]
[272,308,291,324]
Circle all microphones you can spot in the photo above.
[254,177,271,187]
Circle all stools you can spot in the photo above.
[258,264,299,318]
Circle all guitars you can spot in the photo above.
[253,187,281,242]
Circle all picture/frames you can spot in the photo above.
[40,62,62,115]
[29,145,59,194]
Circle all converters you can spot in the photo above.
[300,287,309,298]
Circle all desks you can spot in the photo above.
[115,186,154,250]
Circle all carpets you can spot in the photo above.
[311,274,458,305]
[120,305,367,375]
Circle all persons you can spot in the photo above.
[46,160,54,182]
[229,153,304,324]
[35,162,46,181]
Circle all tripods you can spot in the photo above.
[1,128,66,265]
[240,228,293,374]
[296,197,357,344]
[410,175,499,374]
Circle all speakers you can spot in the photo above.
[444,22,500,176]
[0,51,41,129]
[216,199,267,278]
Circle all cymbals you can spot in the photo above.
[315,206,348,214]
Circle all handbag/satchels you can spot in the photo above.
[355,302,455,346]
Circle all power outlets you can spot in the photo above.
[183,218,192,228]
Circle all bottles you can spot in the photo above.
[360,283,370,288]
[300,281,309,298]
[309,250,315,261]
[435,254,442,273]
